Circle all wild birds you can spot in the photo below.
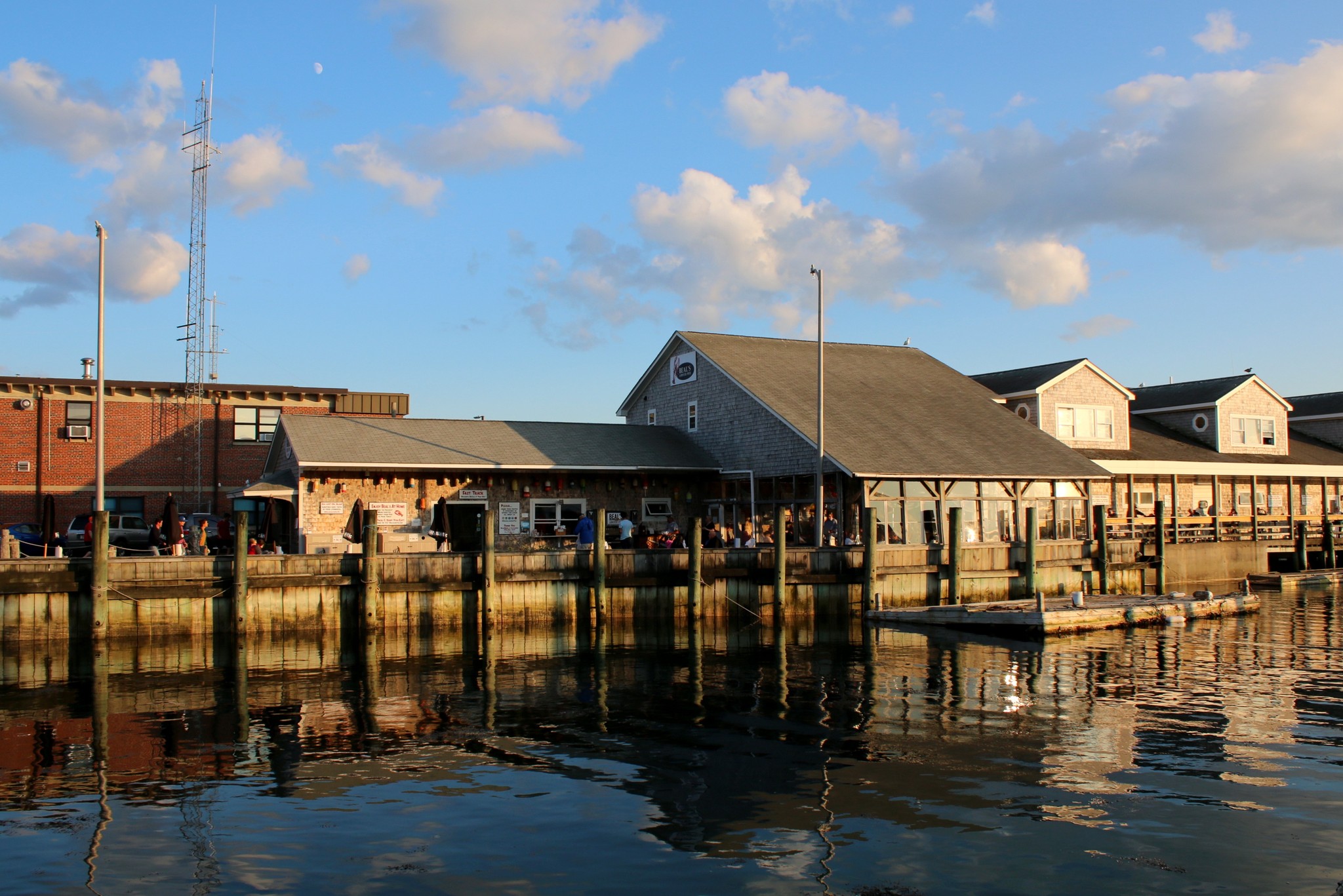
[904,338,911,345]
[1139,383,1143,386]
[1244,368,1253,372]
[811,265,814,276]
[95,220,108,240]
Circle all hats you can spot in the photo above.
[178,516,188,522]
[578,513,584,518]
[88,516,93,521]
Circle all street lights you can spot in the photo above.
[809,260,826,546]
[95,219,112,516]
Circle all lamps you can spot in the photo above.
[19,397,33,410]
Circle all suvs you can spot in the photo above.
[66,513,154,556]
[158,512,263,556]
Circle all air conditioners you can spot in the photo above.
[69,425,91,437]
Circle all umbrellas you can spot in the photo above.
[263,494,283,554]
[343,495,364,544]
[428,494,452,552]
[160,492,184,556]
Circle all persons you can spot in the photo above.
[1126,503,1146,537]
[148,516,188,556]
[247,538,265,555]
[83,516,94,557]
[659,515,686,549]
[216,512,233,555]
[198,519,211,555]
[573,512,594,549]
[701,513,773,548]
[1225,508,1240,538]
[1188,508,1205,543]
[1257,507,1267,533]
[1331,506,1343,538]
[803,508,903,546]
[618,511,634,549]
[1001,530,1065,542]
[1171,507,1187,537]
[1105,507,1120,535]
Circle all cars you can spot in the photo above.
[0,522,67,557]
